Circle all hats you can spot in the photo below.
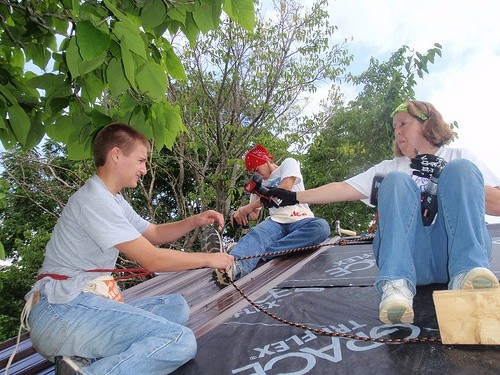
[245,144,274,171]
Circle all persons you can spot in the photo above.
[260,100,500,326]
[202,144,331,289]
[27,123,234,375]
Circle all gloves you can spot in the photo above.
[260,186,299,209]
[410,153,449,179]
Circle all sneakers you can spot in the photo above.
[453,267,500,290]
[54,356,91,375]
[379,279,414,325]
[206,228,236,289]
